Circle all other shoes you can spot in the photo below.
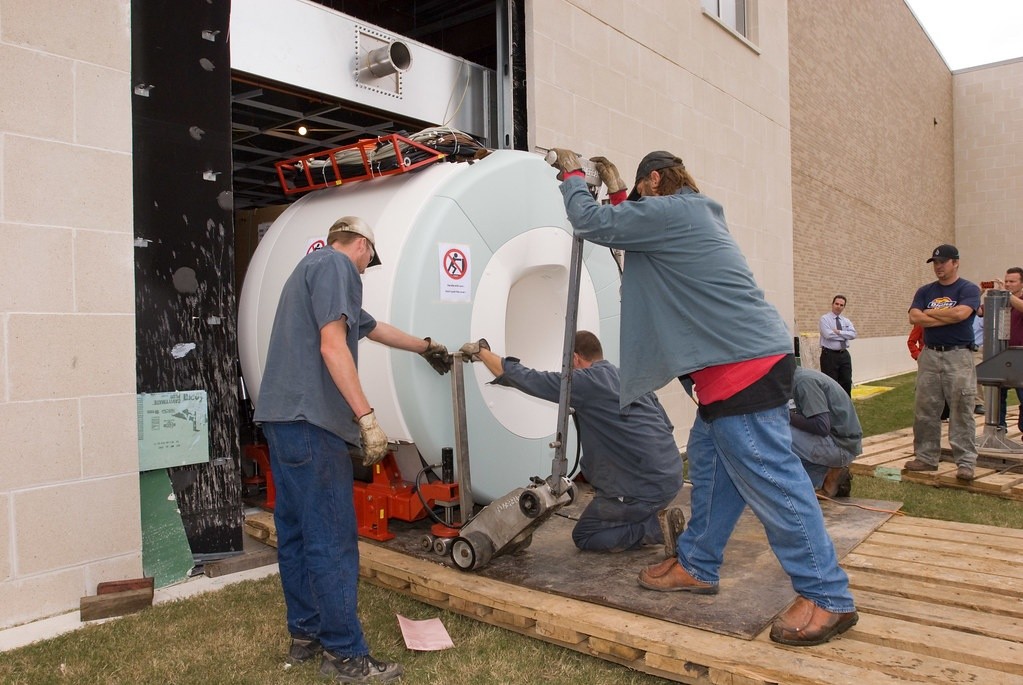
[905,459,938,471]
[815,466,848,500]
[658,507,686,559]
[975,405,985,414]
[957,469,973,479]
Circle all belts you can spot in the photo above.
[925,344,969,352]
[824,348,846,353]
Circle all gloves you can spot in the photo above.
[353,408,388,466]
[545,148,586,180]
[590,157,628,194]
[418,337,451,375]
[459,338,491,363]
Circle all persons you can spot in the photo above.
[973,267,1023,440]
[548,147,859,646]
[789,366,863,497]
[907,323,950,422]
[250,215,451,683]
[971,315,986,415]
[904,244,980,478]
[459,330,685,557]
[819,295,857,398]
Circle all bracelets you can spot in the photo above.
[1010,292,1014,294]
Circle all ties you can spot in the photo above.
[835,317,846,349]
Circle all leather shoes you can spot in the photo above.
[637,557,719,594]
[770,595,858,646]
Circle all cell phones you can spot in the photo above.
[981,281,994,289]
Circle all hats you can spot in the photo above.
[330,216,381,268]
[626,151,683,200]
[927,244,959,263]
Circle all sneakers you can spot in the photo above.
[284,639,322,670]
[317,648,402,685]
